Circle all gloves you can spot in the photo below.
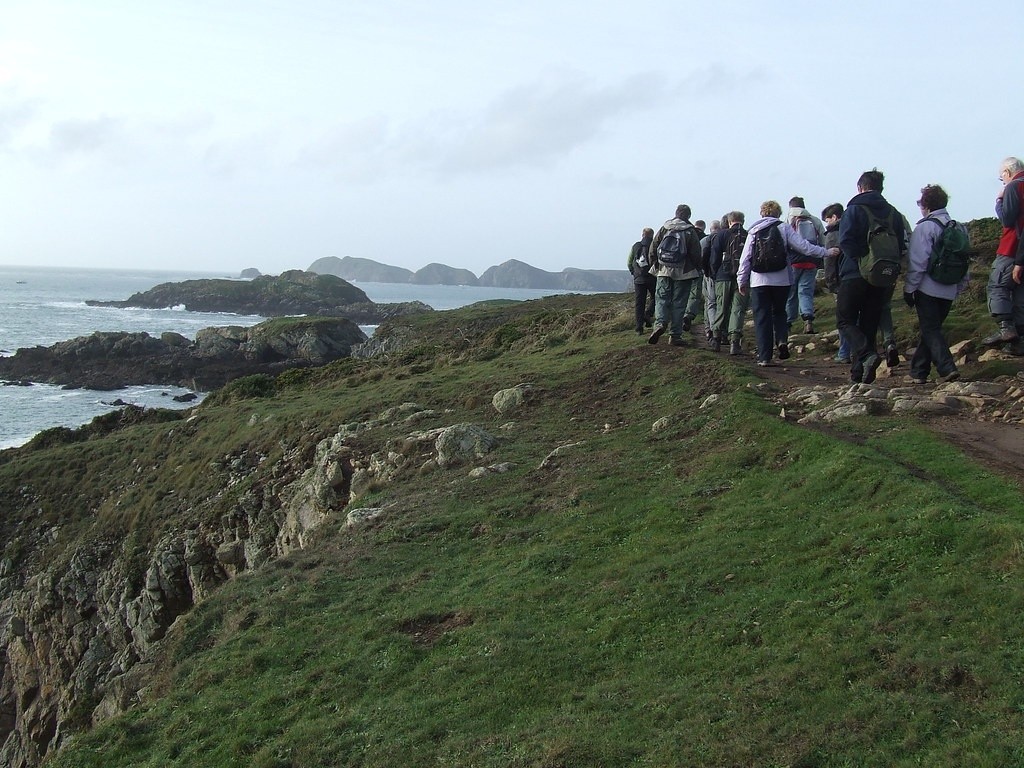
[903,291,915,307]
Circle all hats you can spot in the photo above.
[675,204,691,219]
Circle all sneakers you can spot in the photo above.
[845,379,857,385]
[981,320,1019,346]
[668,335,686,346]
[777,340,790,360]
[886,344,900,367]
[709,339,720,352]
[937,370,961,384]
[730,340,743,354]
[757,359,772,367]
[862,355,883,384]
[902,375,926,384]
[803,320,814,334]
[834,356,851,364]
[649,326,666,344]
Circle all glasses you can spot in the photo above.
[998,170,1006,181]
[917,200,922,206]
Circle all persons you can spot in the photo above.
[822,171,913,384]
[736,201,840,365]
[785,197,828,333]
[628,205,750,355]
[982,157,1024,354]
[902,183,969,385]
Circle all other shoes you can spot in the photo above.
[720,335,729,344]
[636,329,643,335]
[682,316,691,332]
[706,331,712,341]
[645,311,653,327]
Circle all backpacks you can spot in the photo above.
[632,242,654,277]
[916,218,970,285]
[749,221,787,273]
[657,228,691,268]
[790,216,823,269]
[857,204,901,288]
[722,229,748,276]
